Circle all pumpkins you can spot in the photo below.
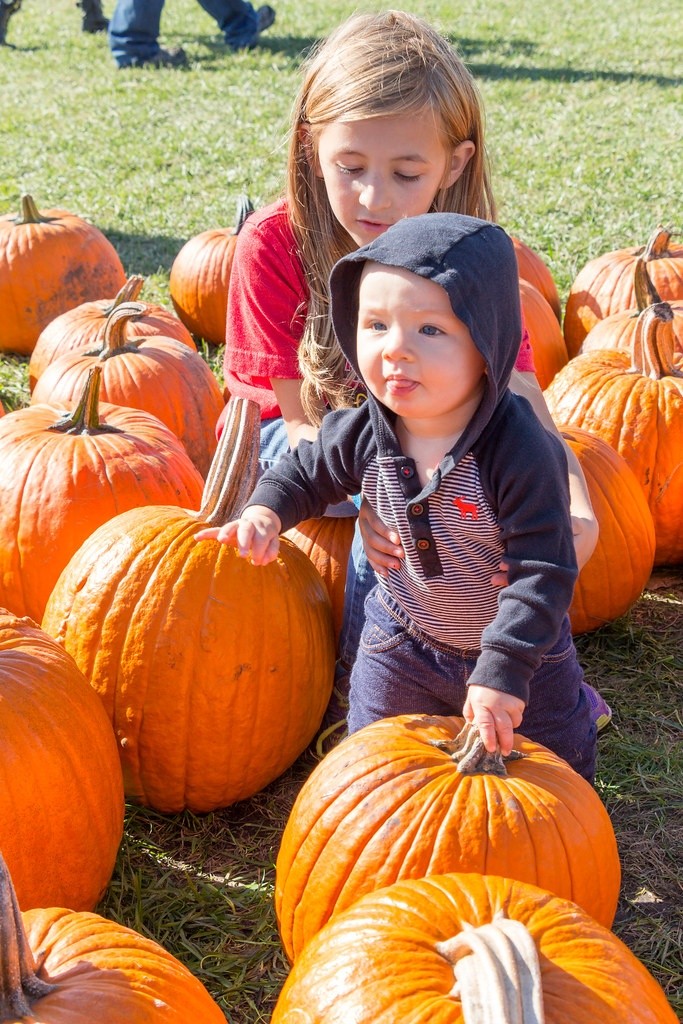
[0,193,356,640]
[0,599,126,914]
[268,871,682,1024]
[507,224,683,637]
[0,844,227,1024]
[273,714,621,968]
[38,397,335,813]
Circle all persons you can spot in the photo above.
[192,210,596,791]
[0,0,276,74]
[214,9,614,765]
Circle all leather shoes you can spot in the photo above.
[226,5,275,53]
[113,46,186,67]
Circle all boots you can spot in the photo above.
[75,0,110,32]
[0,2,18,48]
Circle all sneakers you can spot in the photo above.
[308,675,350,761]
[579,680,612,733]
